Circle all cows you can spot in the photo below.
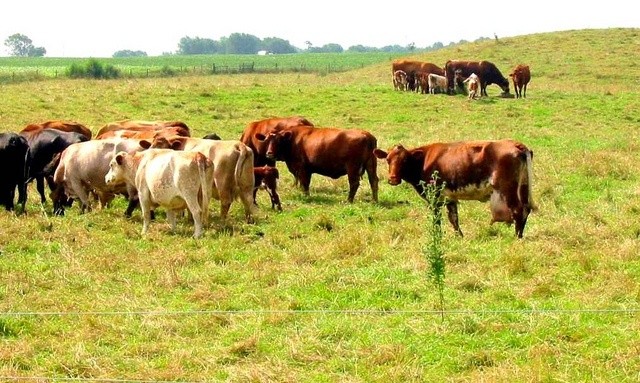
[406,72,415,91]
[371,137,539,240]
[27,127,89,217]
[444,59,510,95]
[252,166,283,213]
[254,124,379,204]
[427,73,448,94]
[138,134,256,225]
[466,72,480,99]
[393,69,409,91]
[413,70,429,94]
[43,137,156,219]
[18,119,92,141]
[103,148,214,240]
[239,115,314,188]
[0,131,29,214]
[391,59,447,94]
[508,64,530,98]
[86,126,189,209]
[96,118,191,137]
[202,133,221,140]
